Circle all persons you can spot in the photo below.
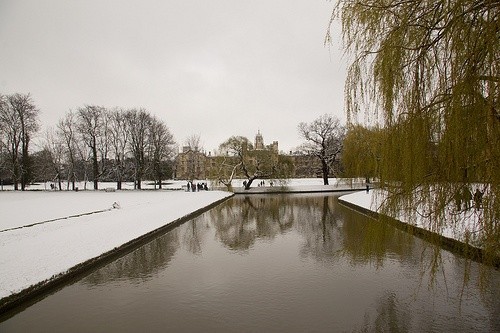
[244,179,274,187]
[453,190,463,212]
[186,182,208,192]
[462,185,473,211]
[473,188,483,211]
